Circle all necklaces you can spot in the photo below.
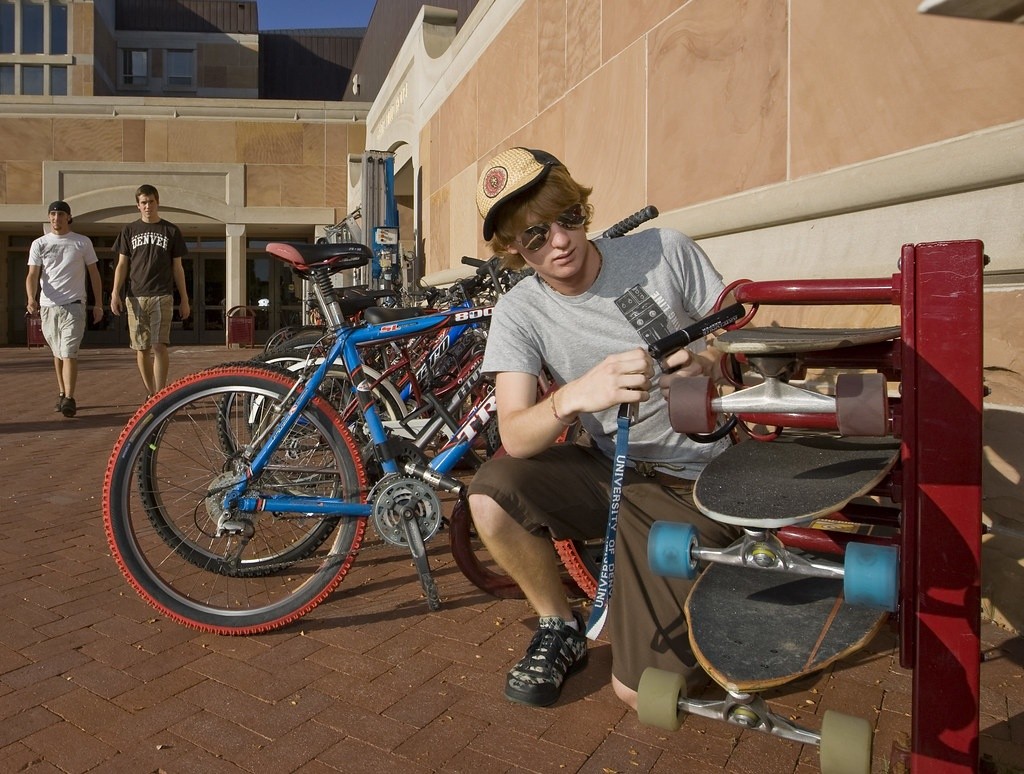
[537,239,603,297]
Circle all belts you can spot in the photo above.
[71,300,82,304]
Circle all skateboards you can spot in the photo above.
[635,535,890,773]
[667,323,901,437]
[644,438,901,614]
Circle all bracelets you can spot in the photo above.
[94,306,104,311]
[551,391,579,426]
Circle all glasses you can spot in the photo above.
[514,202,586,251]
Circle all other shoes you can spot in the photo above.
[146,394,152,400]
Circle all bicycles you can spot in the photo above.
[102,204,666,639]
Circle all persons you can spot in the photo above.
[109,183,191,402]
[465,147,755,709]
[25,200,105,419]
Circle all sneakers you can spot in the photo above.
[502,609,587,704]
[54,392,76,416]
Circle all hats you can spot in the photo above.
[474,148,569,241]
[48,201,73,225]
